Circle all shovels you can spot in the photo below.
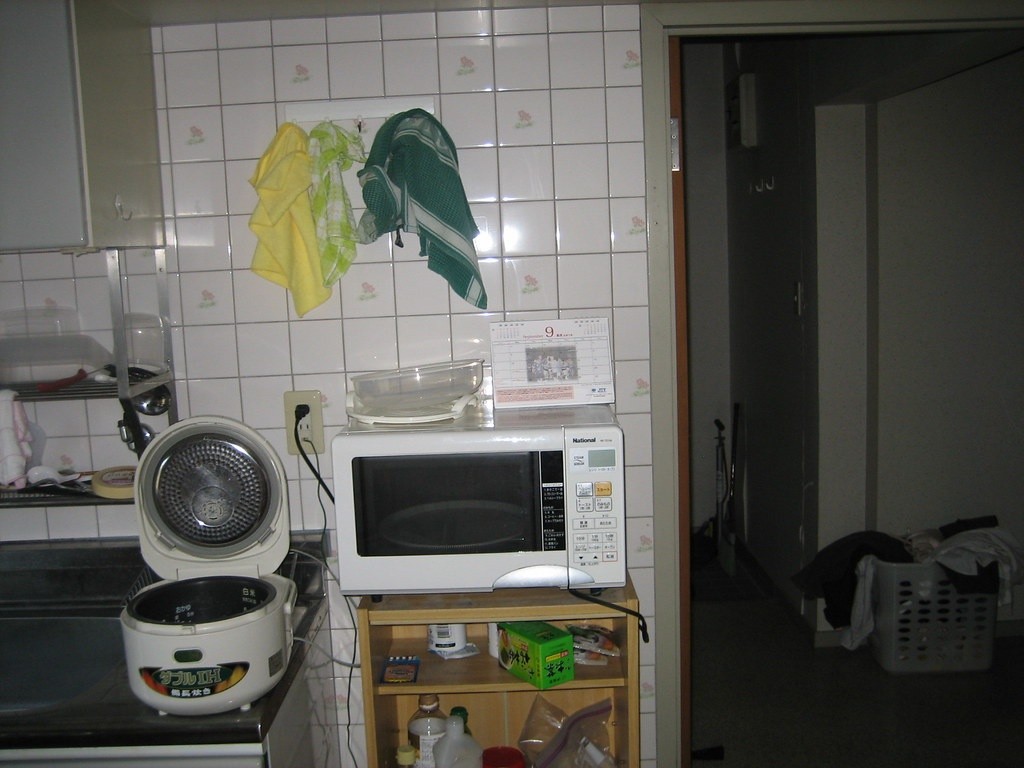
[37,363,157,390]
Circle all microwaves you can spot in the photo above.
[331,404,626,595]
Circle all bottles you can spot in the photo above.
[405,693,448,768]
[432,715,483,768]
[449,706,472,737]
[396,746,416,768]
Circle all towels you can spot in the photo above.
[0,388,38,492]
[243,106,494,319]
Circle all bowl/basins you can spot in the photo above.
[347,358,486,410]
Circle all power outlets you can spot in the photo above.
[284,390,325,456]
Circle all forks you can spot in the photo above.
[26,465,84,493]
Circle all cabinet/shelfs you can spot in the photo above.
[355,569,641,768]
[0,249,180,511]
[0,0,167,258]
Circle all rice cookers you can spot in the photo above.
[120,416,298,717]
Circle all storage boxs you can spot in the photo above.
[869,556,997,674]
[497,621,574,690]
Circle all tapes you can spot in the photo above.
[91,464,135,499]
